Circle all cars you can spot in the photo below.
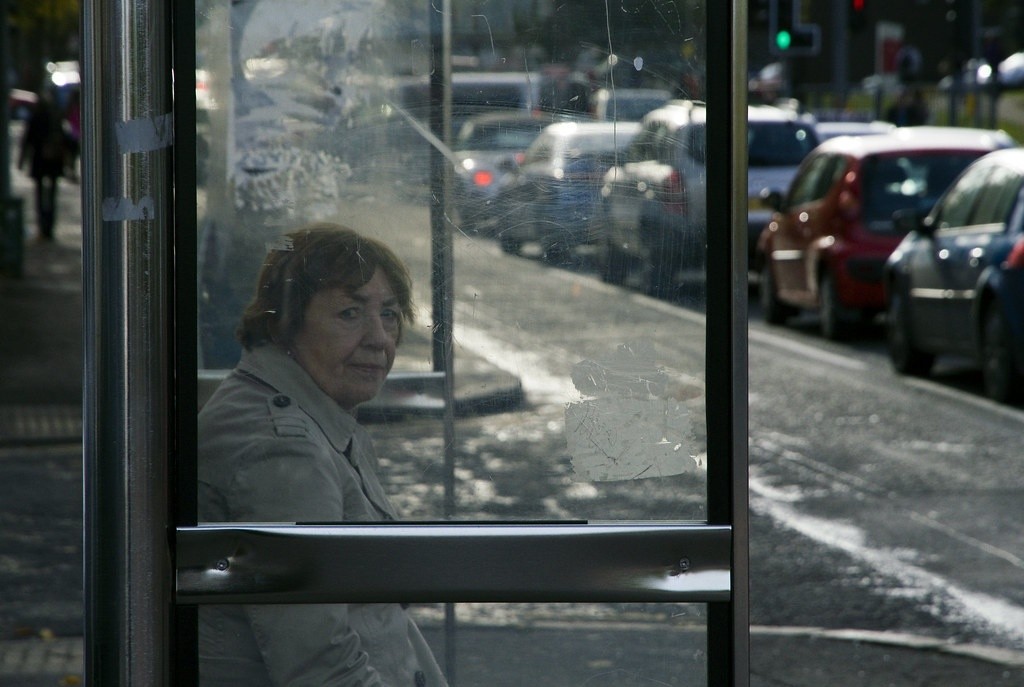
[750,128,1016,341]
[453,112,558,233]
[494,120,643,264]
[879,146,1024,413]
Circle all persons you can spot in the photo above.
[14,76,82,241]
[196,221,452,687]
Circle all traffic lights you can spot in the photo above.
[766,1,823,58]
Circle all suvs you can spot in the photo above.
[597,98,826,300]
